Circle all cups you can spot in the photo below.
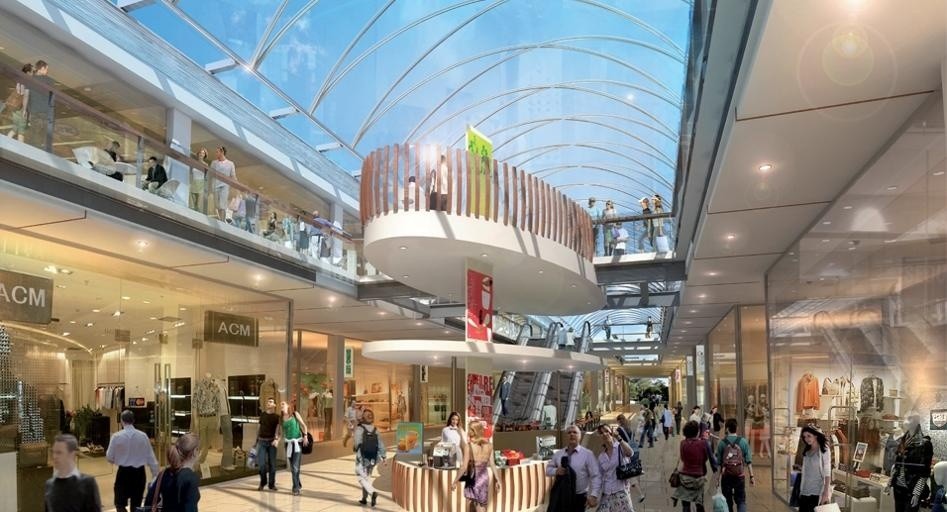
[428,456,433,467]
[405,431,419,451]
[398,438,405,450]
[422,453,427,463]
[495,457,506,465]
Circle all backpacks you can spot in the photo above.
[358,423,378,459]
[620,426,639,461]
[722,436,744,476]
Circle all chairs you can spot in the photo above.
[265,222,285,245]
[71,146,116,177]
[156,179,181,202]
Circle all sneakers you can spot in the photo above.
[371,492,377,506]
[258,485,263,491]
[271,486,277,490]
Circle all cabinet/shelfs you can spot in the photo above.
[351,392,389,405]
[227,374,266,458]
[155,377,192,444]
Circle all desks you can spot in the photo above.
[833,487,877,512]
[832,468,895,512]
[115,161,137,183]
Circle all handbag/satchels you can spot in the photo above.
[136,505,161,512]
[302,432,313,454]
[669,470,681,487]
[616,460,642,480]
[460,463,475,484]
